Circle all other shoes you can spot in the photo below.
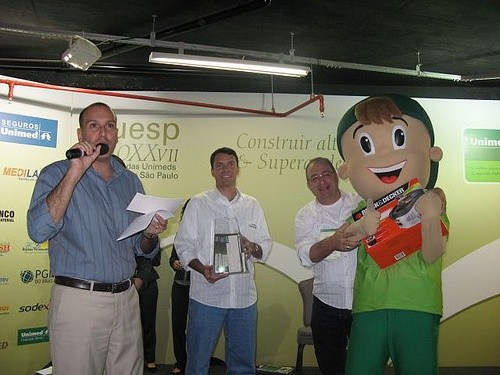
[171,364,187,374]
[147,362,158,373]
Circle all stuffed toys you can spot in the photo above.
[336,93,450,375]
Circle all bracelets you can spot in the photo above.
[253,243,261,259]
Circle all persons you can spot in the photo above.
[294,157,366,375]
[27,102,168,375]
[169,146,272,375]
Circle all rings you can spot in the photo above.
[346,245,350,251]
[155,224,160,229]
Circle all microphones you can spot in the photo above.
[66,143,109,160]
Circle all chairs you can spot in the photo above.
[295,277,315,374]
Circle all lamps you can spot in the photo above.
[149,13,310,77]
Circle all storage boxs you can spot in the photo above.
[351,178,449,268]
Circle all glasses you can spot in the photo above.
[309,172,332,183]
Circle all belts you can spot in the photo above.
[54,275,135,293]
